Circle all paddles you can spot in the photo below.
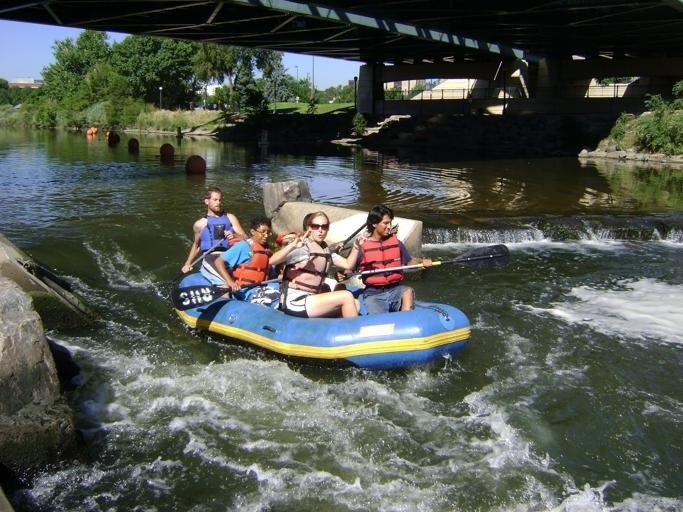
[172,278,279,311]
[346,245,509,278]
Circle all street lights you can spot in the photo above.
[159,87,163,109]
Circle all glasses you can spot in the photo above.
[255,229,273,238]
[307,222,329,230]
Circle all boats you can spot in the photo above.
[175,272,471,370]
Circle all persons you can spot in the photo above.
[276,212,345,282]
[269,212,361,318]
[214,215,281,310]
[335,205,432,314]
[181,186,249,299]
